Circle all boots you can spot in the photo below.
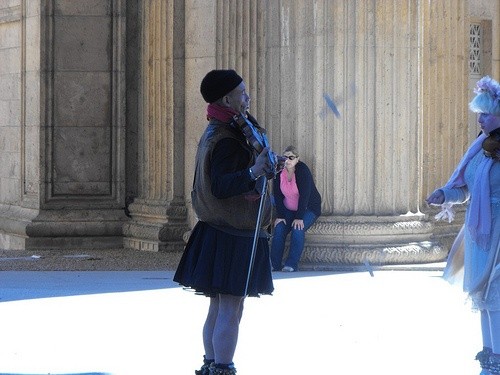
[208,362,237,375]
[194,354,215,375]
[474,346,493,375]
[487,352,500,375]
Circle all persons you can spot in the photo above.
[427,75,500,375]
[270,145,321,272]
[172,70,286,375]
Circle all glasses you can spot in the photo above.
[283,154,299,160]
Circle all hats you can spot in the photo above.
[200,69,243,104]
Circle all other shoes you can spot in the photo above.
[282,266,295,272]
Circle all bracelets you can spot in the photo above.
[250,168,258,181]
